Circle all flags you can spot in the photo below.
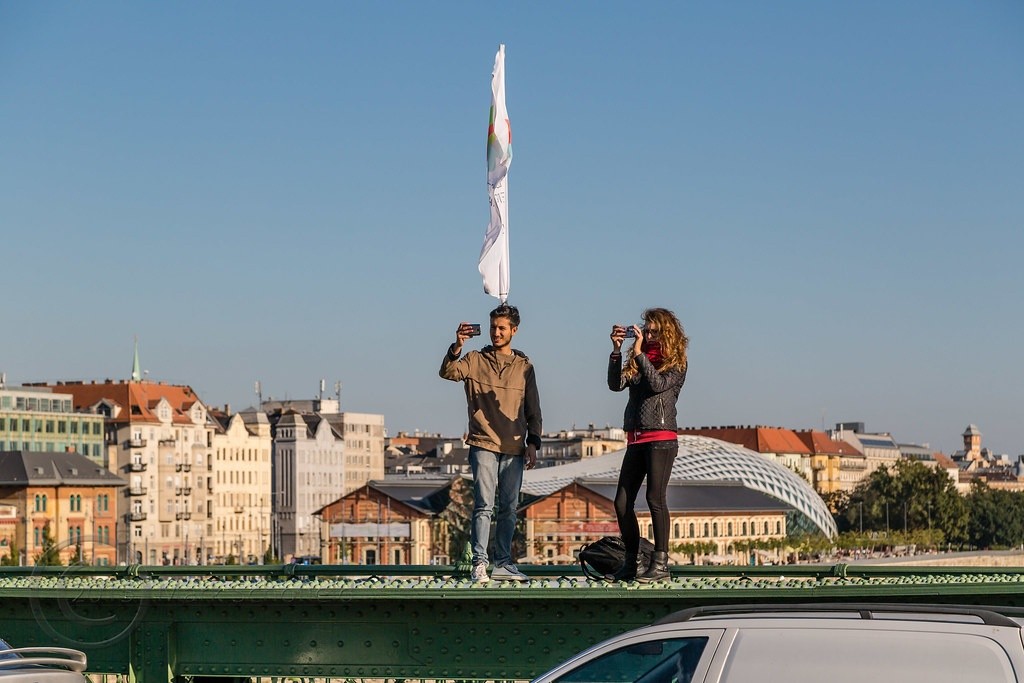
[476,48,512,301]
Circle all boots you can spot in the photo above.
[636,550,671,583]
[599,554,640,582]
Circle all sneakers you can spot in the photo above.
[470,560,490,584]
[489,563,529,583]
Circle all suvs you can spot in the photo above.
[529,600,1024,683]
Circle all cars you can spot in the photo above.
[0,639,91,683]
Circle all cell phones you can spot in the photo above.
[621,326,636,338]
[464,324,481,336]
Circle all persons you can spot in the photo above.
[438,301,542,582]
[607,307,691,584]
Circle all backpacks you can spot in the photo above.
[577,533,659,581]
